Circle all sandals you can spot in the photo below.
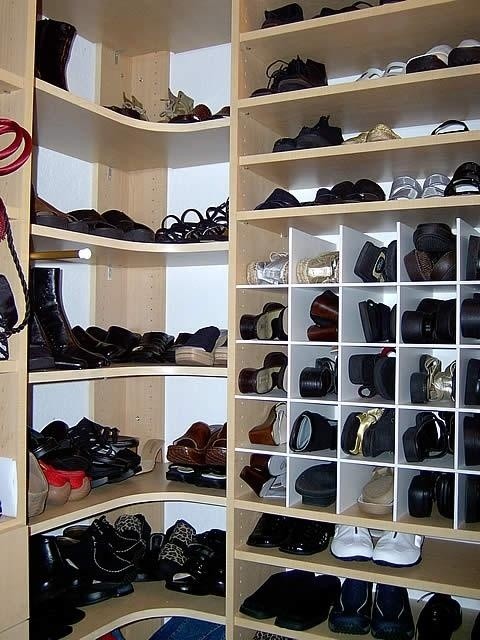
[310,1,374,19]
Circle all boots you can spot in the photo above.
[155,87,195,124]
[35,16,77,95]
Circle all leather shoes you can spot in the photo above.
[255,161,479,211]
[237,218,480,568]
[239,568,316,619]
[101,93,148,121]
[168,99,211,126]
[418,591,462,640]
[29,417,143,517]
[470,611,480,640]
[204,103,230,120]
[29,181,231,371]
[30,513,225,638]
[274,574,341,631]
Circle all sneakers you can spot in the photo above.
[370,583,415,640]
[327,577,373,636]
[259,2,307,30]
[273,115,345,152]
[251,55,328,96]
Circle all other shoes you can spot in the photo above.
[342,123,401,144]
[355,39,479,83]
[432,119,470,135]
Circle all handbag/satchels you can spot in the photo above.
[0,274,20,342]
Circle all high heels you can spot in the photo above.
[135,438,165,476]
